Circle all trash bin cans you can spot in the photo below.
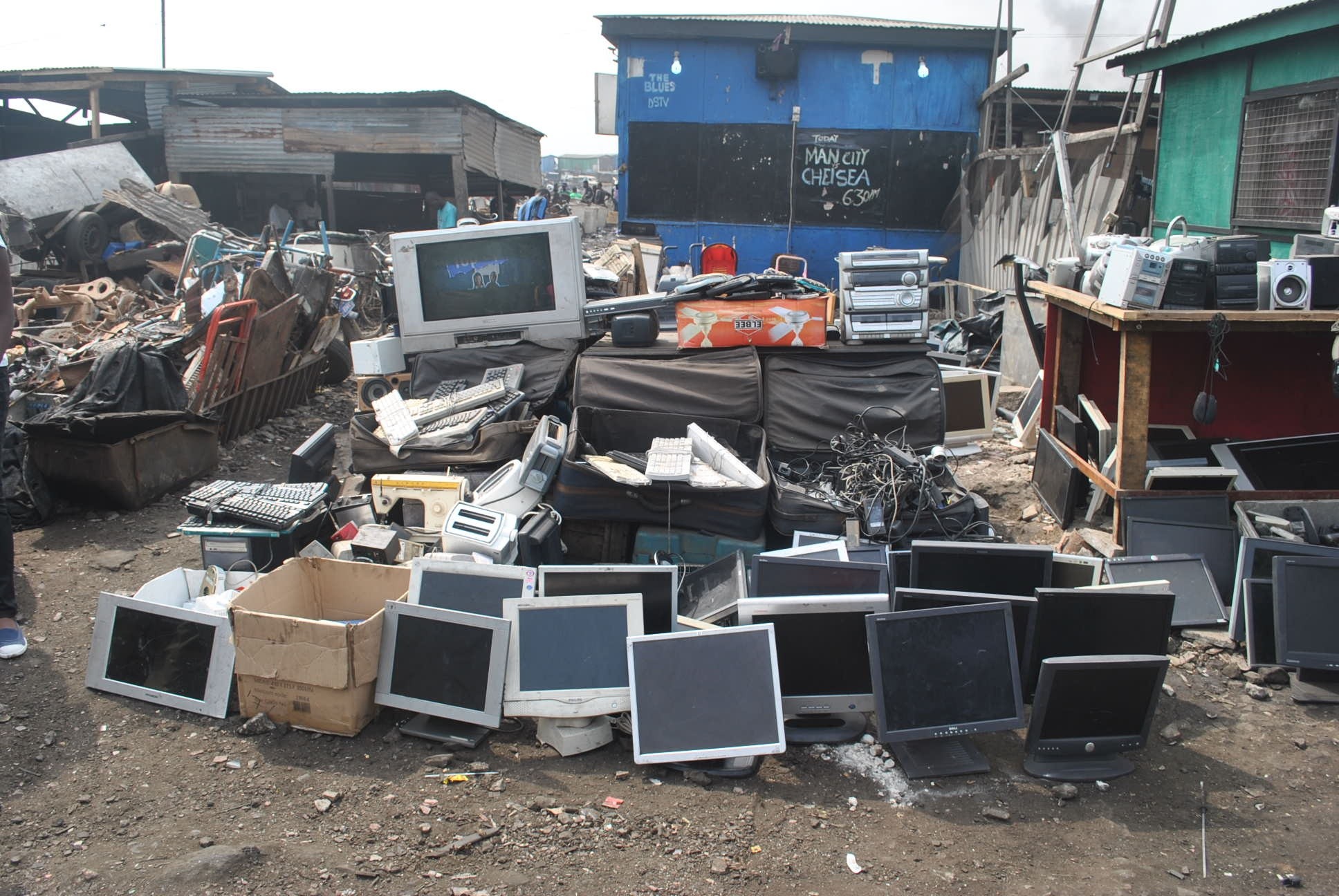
[23,411,220,512]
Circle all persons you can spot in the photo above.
[473,271,498,289]
[0,237,26,658]
[425,179,619,230]
[268,189,321,236]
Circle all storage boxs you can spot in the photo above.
[226,556,411,737]
[675,296,829,351]
[31,419,219,511]
[132,566,268,618]
[350,337,406,375]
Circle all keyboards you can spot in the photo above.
[661,271,829,303]
[580,420,765,490]
[582,244,632,297]
[371,363,526,444]
[177,481,329,539]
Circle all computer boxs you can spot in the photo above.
[200,513,322,573]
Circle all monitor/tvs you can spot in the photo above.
[288,423,342,508]
[1030,393,1339,704]
[388,216,589,356]
[1011,370,1045,449]
[85,591,235,719]
[926,351,1002,457]
[373,530,1178,778]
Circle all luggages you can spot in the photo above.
[544,346,772,540]
[350,337,580,473]
[763,354,977,547]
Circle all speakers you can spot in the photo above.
[350,337,406,376]
[355,372,411,412]
[1256,254,1339,311]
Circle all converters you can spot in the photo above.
[887,520,910,551]
[883,445,921,471]
[863,496,886,539]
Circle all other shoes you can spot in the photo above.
[0,619,27,659]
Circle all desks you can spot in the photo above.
[1026,280,1339,544]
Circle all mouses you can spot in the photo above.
[1192,391,1218,426]
